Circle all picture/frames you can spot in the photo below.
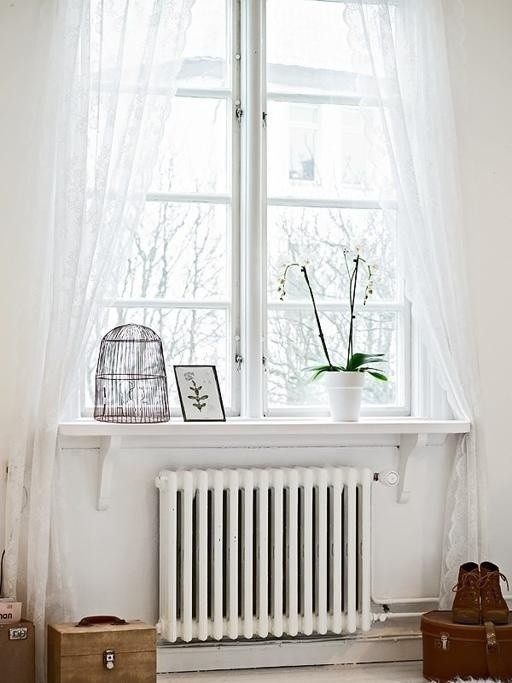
[172,364,226,421]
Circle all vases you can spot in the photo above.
[323,371,365,421]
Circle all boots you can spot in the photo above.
[480,562,509,624]
[451,562,481,623]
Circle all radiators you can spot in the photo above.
[152,467,377,645]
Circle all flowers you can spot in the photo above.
[275,246,391,381]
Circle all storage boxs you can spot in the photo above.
[1,620,157,682]
